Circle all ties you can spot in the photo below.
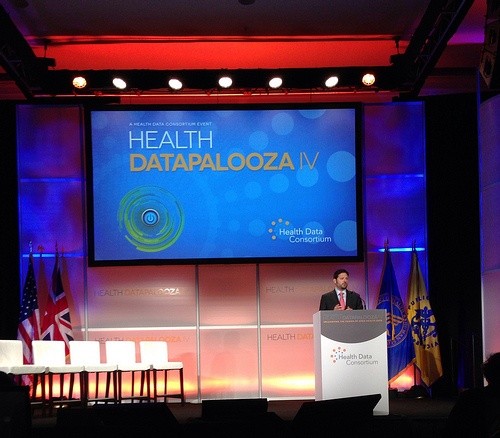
[340,292,346,310]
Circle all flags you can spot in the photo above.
[373,240,423,392]
[41,247,75,356]
[404,241,444,387]
[13,251,43,386]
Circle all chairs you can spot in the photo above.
[0,341,184,417]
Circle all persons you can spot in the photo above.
[319,269,364,310]
[445,352,500,438]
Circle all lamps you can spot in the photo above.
[267,74,282,90]
[216,71,232,89]
[320,72,338,88]
[168,77,184,91]
[361,70,377,87]
[112,75,131,92]
[71,72,88,90]
